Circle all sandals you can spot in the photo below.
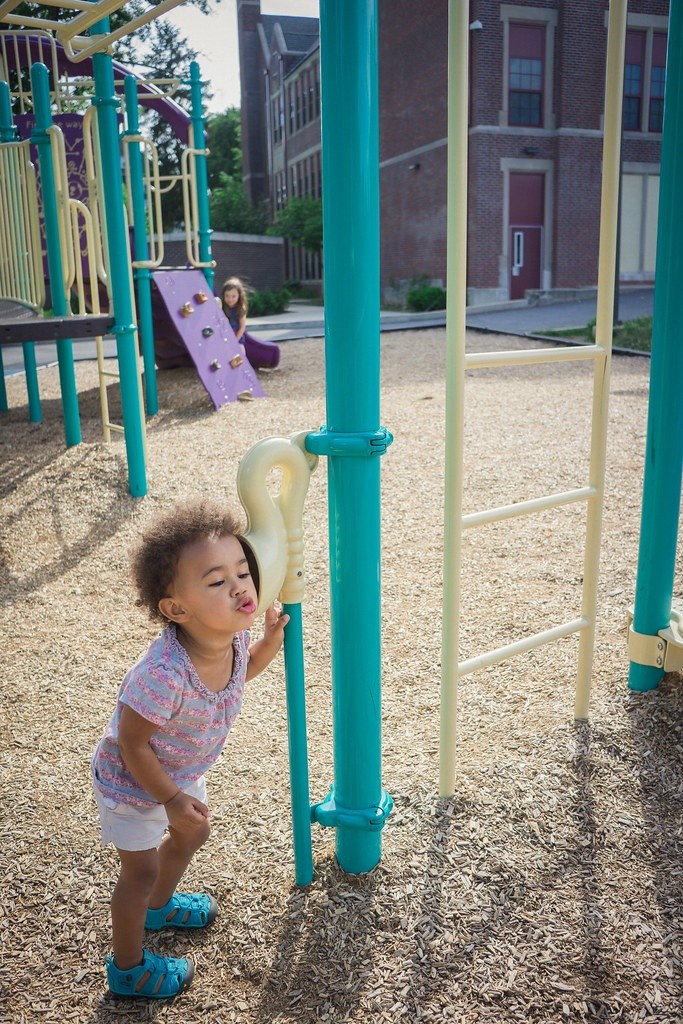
[144,891,217,932]
[107,948,194,998]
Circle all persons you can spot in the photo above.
[91,509,290,999]
[221,276,247,358]
